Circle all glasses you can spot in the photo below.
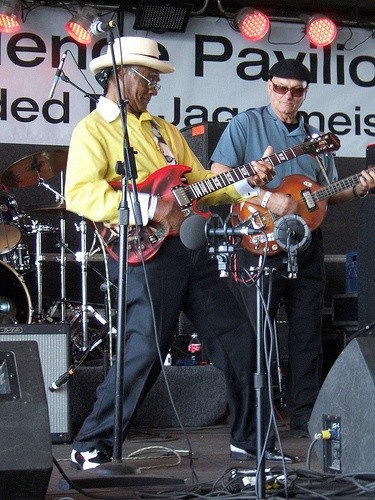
[270,80,307,97]
[131,65,161,91]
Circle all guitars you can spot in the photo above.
[97,132,341,266]
[229,165,375,257]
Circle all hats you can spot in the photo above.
[267,59,313,83]
[88,37,175,76]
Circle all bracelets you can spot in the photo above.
[352,185,368,199]
[261,191,272,208]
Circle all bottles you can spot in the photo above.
[164,348,172,366]
[187,333,202,365]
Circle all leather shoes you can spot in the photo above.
[230,444,301,463]
[290,423,311,438]
[70,448,113,471]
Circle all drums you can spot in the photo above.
[0,260,33,325]
[2,243,31,271]
[0,191,23,256]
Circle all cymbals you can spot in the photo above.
[0,149,69,191]
[31,202,73,214]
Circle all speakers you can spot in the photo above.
[0,340,52,500]
[179,120,233,219]
[307,336,375,479]
[0,325,72,444]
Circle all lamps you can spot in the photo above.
[133,0,192,33]
[302,13,337,47]
[0,0,23,32]
[66,7,102,44]
[216,0,269,40]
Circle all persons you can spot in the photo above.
[65,36,301,472]
[208,56,375,436]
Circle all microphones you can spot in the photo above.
[89,20,116,35]
[180,212,263,250]
[48,53,67,99]
[48,370,75,392]
